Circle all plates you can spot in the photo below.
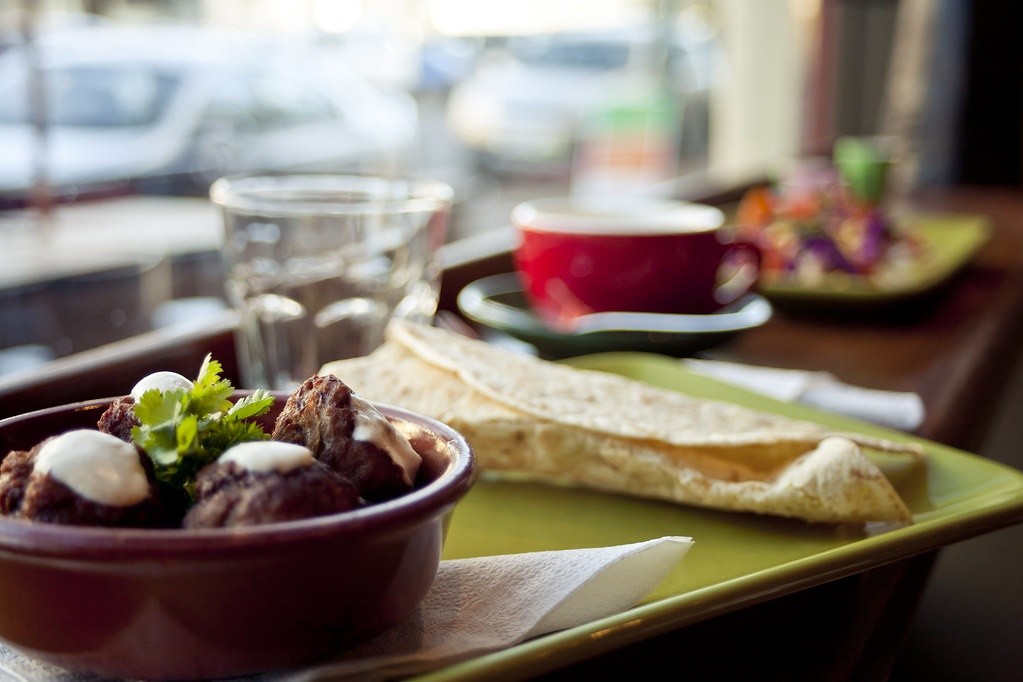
[452,271,770,356]
[411,349,1023,682]
[758,212,995,308]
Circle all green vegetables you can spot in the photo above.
[131,352,277,500]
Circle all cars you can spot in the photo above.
[1,33,701,213]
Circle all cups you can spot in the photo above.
[208,170,456,393]
[509,195,725,319]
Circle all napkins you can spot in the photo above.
[0,532,695,682]
[676,357,926,432]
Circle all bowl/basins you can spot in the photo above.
[0,387,479,682]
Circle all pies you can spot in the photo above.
[317,316,922,524]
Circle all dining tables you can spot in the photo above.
[384,181,1023,682]
